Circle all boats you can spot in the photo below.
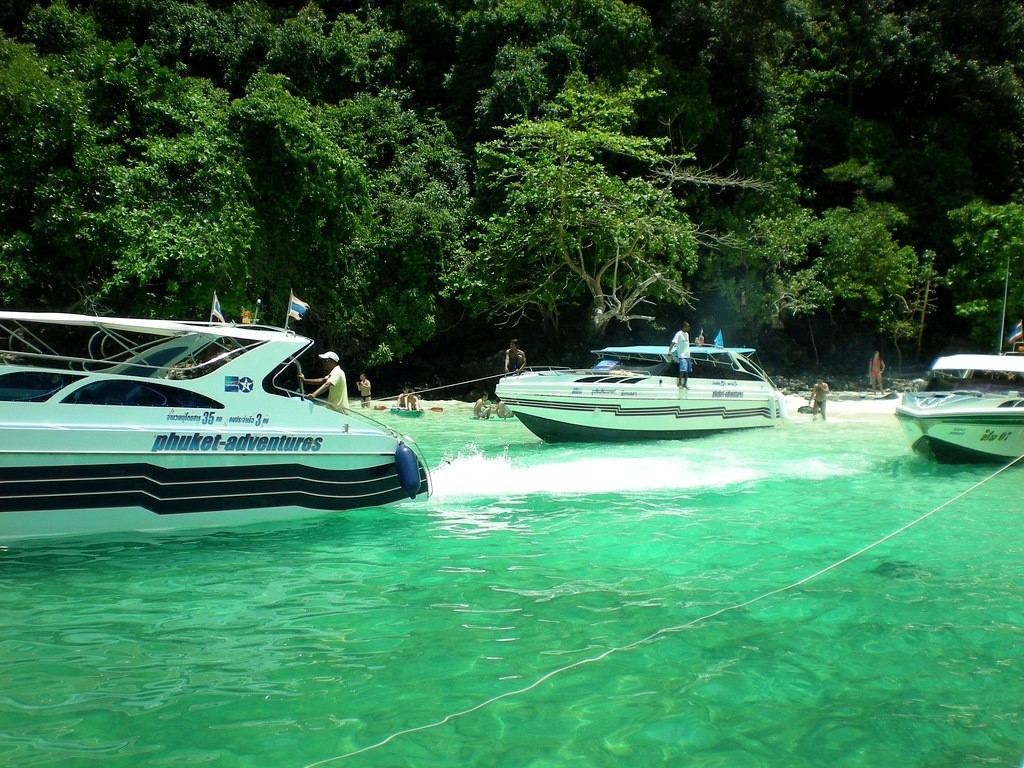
[492,330,788,445]
[896,255,1024,470]
[0,305,436,541]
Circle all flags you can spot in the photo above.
[715,330,723,348]
[212,295,224,323]
[1008,322,1022,344]
[288,295,310,320]
[699,330,704,343]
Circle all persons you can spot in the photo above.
[668,321,691,390]
[869,351,885,396]
[297,351,349,416]
[356,373,371,408]
[474,392,514,420]
[505,339,526,374]
[809,377,829,420]
[397,387,420,411]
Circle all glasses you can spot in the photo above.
[323,359,331,362]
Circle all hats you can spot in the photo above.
[318,351,339,362]
